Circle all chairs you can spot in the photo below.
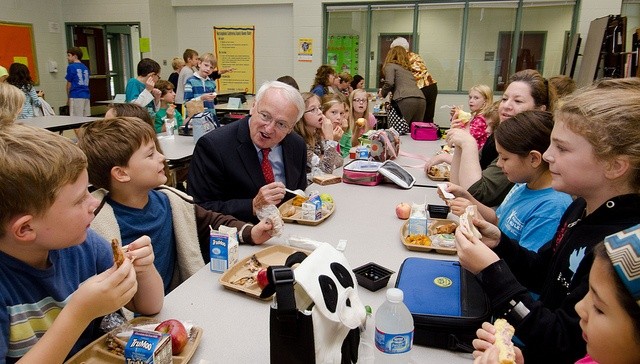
[166,154,193,196]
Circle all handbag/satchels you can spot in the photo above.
[183,111,220,145]
[394,257,496,353]
[411,122,443,141]
[343,159,416,190]
[260,242,367,364]
[368,126,400,162]
[387,99,410,136]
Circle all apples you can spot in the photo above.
[357,118,365,125]
[155,318,188,356]
[396,203,412,219]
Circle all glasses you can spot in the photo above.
[157,74,161,79]
[353,98,368,102]
[304,107,323,113]
[257,102,293,130]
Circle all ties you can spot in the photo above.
[261,148,274,184]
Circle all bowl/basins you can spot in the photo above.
[426,204,451,218]
[352,263,396,291]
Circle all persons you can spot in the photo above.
[1,125,165,364]
[167,58,185,93]
[196,57,235,80]
[481,100,502,138]
[294,92,344,187]
[390,37,438,123]
[0,83,26,125]
[377,45,426,133]
[64,47,91,117]
[450,84,493,152]
[321,93,344,155]
[445,68,577,207]
[309,65,364,96]
[472,224,640,364]
[78,117,284,321]
[104,103,172,187]
[174,48,199,104]
[125,57,162,128]
[456,78,640,364]
[437,110,574,254]
[151,79,190,133]
[183,53,218,143]
[6,63,45,120]
[187,81,307,225]
[348,88,377,147]
[337,92,352,159]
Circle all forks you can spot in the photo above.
[282,186,305,197]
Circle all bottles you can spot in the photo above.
[371,288,415,364]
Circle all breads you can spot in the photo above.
[494,318,516,364]
[460,205,483,242]
[314,174,341,185]
[457,110,472,124]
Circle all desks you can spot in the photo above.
[17,115,104,136]
[157,132,202,190]
[95,99,126,106]
[213,100,253,125]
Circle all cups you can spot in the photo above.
[165,118,175,137]
